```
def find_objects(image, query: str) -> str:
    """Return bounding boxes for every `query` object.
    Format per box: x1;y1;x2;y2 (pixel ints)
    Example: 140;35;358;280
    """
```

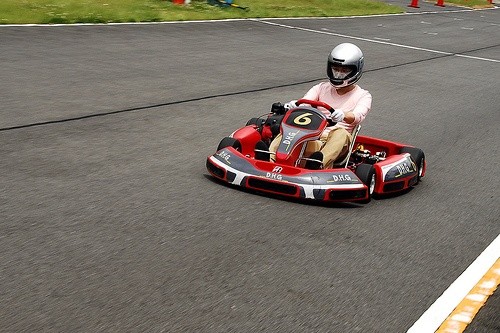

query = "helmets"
326;42;364;88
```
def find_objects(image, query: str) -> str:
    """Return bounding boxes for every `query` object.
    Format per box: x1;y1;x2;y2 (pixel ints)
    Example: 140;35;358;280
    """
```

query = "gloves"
328;108;345;122
288;99;304;108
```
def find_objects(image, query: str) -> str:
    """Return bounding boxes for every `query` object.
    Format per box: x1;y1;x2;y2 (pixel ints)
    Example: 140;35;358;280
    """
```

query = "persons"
254;43;371;170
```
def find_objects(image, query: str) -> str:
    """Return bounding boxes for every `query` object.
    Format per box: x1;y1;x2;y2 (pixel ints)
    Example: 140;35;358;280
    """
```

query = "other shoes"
255;140;270;161
305;151;323;169
